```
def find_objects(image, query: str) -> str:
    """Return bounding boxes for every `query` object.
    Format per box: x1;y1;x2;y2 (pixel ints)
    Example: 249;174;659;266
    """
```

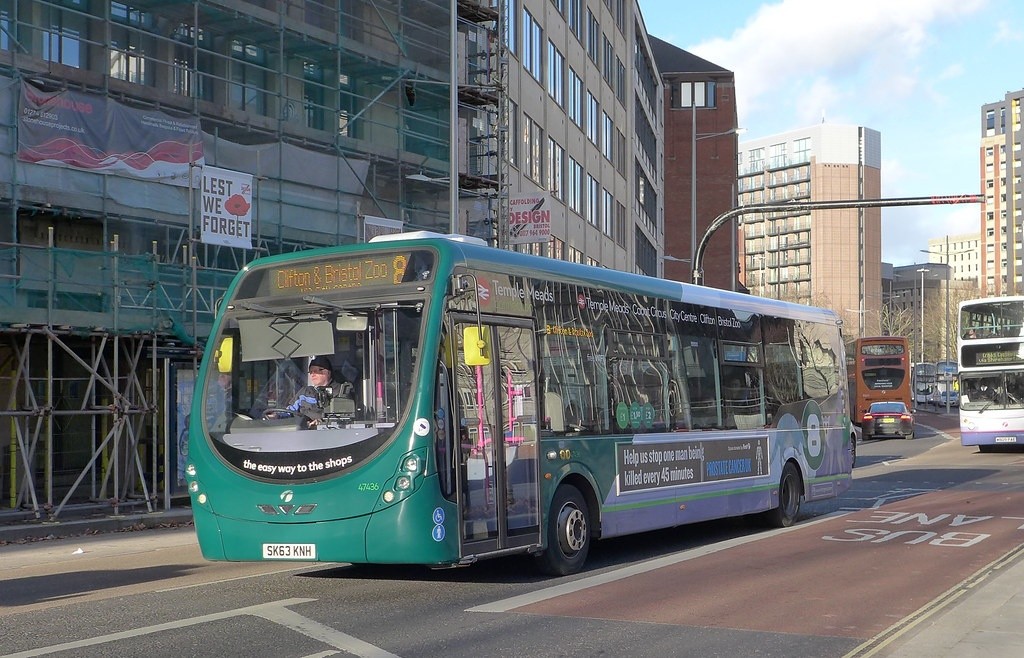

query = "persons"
975;382;995;401
263;357;358;427
961;326;976;338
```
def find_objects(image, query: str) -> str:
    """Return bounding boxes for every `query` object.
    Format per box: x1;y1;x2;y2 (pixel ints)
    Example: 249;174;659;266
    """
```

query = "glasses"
307;368;326;374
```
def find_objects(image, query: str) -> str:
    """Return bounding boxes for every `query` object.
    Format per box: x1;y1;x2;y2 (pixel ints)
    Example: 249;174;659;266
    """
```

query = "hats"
308;356;333;371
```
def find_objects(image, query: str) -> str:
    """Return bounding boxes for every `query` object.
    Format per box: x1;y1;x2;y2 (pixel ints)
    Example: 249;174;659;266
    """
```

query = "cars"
861;401;914;440
938;391;959;408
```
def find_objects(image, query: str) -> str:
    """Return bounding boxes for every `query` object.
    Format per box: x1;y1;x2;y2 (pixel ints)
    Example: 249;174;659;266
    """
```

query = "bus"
911;362;936;403
956;295;1024;452
845;337;911;425
184;231;851;576
936;361;959;395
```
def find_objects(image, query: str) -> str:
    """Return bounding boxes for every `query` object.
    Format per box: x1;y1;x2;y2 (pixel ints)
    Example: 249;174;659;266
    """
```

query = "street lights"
920;235;974;414
